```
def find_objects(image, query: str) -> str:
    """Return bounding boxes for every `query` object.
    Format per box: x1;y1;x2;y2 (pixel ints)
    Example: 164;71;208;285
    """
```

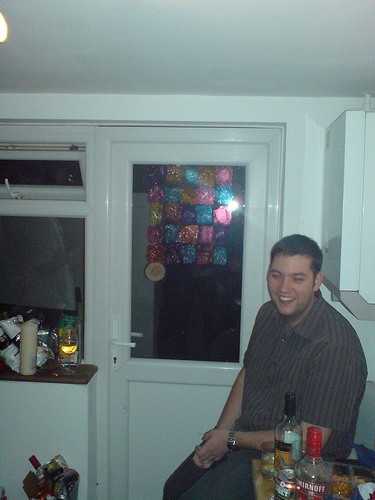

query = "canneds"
274;469;299;500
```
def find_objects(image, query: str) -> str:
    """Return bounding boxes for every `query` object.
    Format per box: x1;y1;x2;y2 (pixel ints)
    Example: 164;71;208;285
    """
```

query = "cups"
261;441;274;478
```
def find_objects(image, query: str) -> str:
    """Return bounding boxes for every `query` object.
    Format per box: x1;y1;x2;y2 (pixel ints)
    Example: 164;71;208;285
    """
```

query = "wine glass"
59;333;78;374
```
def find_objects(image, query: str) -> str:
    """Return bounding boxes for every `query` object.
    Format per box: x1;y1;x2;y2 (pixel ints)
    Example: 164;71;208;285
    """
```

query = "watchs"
227;430;236;452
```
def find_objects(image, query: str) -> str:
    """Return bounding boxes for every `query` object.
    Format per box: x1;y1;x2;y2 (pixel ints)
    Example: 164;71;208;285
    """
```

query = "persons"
161;233;369;500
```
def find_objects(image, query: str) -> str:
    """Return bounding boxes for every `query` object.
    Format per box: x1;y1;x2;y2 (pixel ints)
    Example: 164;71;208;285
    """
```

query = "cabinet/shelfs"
321;110;375;305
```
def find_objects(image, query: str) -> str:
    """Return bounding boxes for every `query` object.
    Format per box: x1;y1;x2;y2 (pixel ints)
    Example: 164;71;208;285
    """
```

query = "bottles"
295;427;332;500
273;392;303;488
